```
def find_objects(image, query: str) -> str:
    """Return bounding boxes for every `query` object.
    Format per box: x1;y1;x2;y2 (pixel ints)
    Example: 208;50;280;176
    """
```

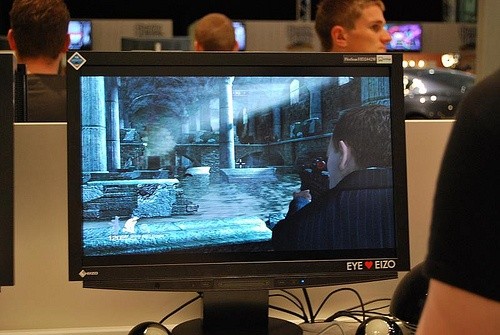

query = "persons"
315;0;392;52
8;0;71;122
193;13;238;51
413;72;500;335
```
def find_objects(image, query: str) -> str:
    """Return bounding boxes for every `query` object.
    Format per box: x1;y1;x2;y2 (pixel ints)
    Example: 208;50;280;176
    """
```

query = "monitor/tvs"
65;50;411;335
231;20;248;51
383;23;423;52
67;18;93;50
